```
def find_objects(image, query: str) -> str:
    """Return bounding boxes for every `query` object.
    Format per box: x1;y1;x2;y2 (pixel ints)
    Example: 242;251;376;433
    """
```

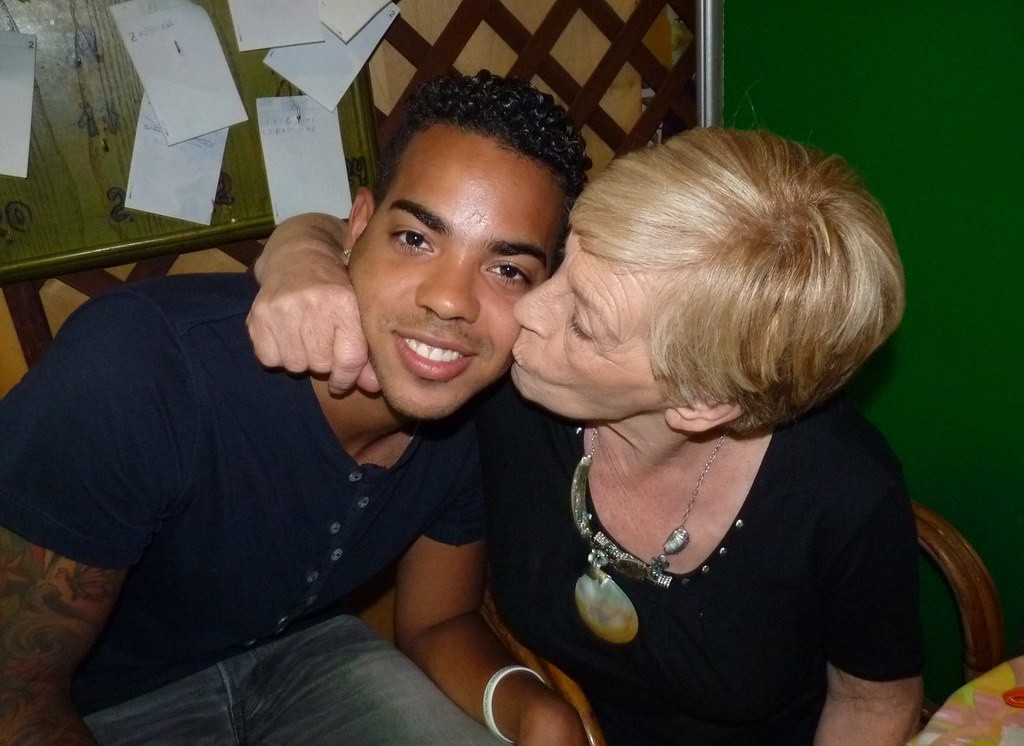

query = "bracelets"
481;663;548;744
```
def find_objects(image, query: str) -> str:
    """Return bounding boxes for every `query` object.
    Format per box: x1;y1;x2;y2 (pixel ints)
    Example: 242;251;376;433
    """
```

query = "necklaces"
568;423;732;646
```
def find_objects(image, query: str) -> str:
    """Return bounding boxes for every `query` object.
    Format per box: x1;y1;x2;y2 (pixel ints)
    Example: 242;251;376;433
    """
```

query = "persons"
0;68;596;746
244;122;928;746
